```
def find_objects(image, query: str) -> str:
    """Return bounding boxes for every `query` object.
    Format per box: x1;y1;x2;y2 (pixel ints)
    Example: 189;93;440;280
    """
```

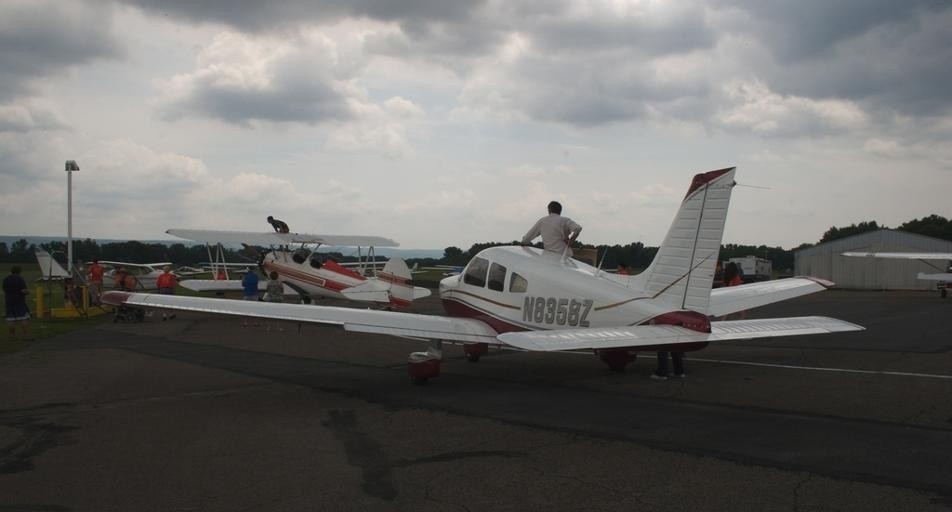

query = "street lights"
66;160;80;275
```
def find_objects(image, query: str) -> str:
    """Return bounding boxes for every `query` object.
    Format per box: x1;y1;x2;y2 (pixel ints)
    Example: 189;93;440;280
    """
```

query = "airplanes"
36;251;177;290
99;166;866;386
167;229;431;310
842;251;952;297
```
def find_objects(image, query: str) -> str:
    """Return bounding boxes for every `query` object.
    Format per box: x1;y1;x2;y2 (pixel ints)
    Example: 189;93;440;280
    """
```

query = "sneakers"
670;372;685;378
650;374;669;381
161;313;175;321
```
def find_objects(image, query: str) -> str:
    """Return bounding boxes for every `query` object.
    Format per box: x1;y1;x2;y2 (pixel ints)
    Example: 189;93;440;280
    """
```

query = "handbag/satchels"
262;291;270;302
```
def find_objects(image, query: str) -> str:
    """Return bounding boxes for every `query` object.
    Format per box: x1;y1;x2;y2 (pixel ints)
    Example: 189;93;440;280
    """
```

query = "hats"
247;266;255;271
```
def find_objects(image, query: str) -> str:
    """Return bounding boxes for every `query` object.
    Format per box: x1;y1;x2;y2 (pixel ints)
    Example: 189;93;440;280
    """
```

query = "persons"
648;344;686;381
241;263;262;327
267;215;290;253
265;269;286;332
722;261;745;320
113;267;135;317
2;264;35;342
521;200;583;261
87;256;104;306
156;266;178;320
614;262;627;274
